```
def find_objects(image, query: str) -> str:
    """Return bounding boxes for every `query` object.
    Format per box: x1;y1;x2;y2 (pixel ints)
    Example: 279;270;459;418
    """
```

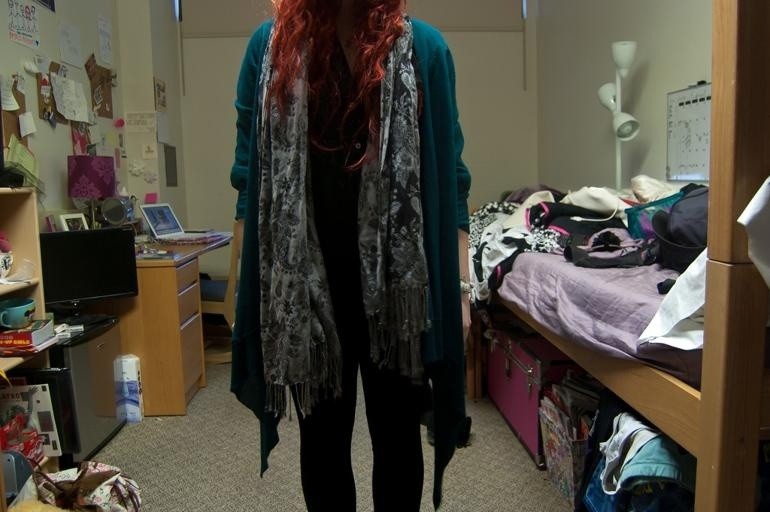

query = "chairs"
202;219;246;367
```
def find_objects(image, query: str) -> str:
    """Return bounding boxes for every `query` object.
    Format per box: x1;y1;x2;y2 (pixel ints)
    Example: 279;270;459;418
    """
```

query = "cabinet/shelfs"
0;185;85;512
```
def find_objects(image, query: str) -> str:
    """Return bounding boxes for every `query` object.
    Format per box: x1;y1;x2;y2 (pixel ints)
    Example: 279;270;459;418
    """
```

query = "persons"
226;1;472;512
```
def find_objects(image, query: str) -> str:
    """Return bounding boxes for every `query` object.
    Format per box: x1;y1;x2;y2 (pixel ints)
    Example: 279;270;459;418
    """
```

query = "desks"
90;233;231;418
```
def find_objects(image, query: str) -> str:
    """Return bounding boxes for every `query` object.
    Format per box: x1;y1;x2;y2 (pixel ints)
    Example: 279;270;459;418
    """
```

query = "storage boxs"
482;323;600;512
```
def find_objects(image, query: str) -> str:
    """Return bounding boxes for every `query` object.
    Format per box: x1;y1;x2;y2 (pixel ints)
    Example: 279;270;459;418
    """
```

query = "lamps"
597;40;638;192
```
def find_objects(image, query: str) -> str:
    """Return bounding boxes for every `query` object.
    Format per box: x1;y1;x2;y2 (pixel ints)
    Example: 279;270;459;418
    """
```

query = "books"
0;335;59;353
120;354;145;422
0;319;55;346
114;357;127;423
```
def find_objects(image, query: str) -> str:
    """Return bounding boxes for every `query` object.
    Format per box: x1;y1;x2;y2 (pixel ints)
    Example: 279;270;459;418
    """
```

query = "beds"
455;195;770;512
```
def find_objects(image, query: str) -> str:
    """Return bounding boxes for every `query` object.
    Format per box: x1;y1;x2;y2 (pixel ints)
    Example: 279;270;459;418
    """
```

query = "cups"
0;297;36;330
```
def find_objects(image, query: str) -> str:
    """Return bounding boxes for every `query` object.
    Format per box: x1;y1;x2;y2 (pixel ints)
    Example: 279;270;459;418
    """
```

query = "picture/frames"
45;211;90;233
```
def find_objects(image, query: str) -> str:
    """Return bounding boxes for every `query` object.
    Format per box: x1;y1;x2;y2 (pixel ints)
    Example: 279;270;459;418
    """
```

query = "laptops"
139;203;208;241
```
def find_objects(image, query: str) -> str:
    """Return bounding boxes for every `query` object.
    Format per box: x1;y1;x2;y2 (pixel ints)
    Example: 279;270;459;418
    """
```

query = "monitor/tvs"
40;227;138;326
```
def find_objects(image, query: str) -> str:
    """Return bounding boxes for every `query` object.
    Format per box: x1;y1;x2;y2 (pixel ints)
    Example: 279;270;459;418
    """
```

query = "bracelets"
460;278;473;294
